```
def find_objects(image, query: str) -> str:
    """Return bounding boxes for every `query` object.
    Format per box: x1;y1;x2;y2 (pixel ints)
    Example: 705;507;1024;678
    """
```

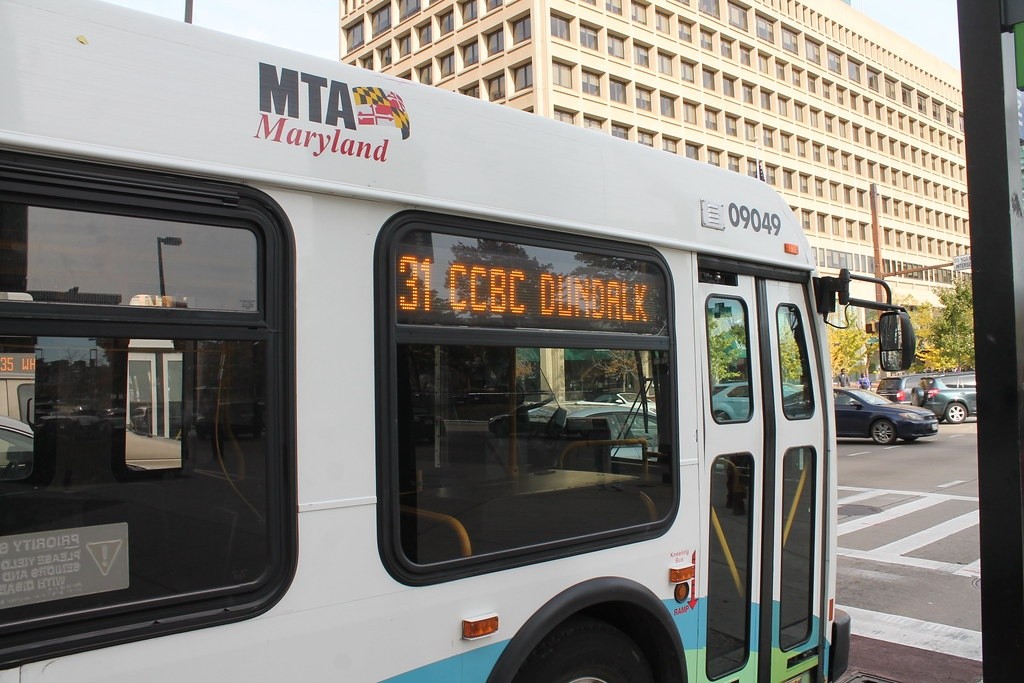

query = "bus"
0;339;185;469
2;0;916;681
515;307;749;404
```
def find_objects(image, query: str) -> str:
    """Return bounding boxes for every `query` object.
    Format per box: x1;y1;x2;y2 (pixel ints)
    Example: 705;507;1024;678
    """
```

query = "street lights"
156;235;182;298
36;347;43;359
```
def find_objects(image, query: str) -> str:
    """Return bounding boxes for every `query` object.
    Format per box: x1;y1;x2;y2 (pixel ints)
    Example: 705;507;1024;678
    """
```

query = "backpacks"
860;378;869;390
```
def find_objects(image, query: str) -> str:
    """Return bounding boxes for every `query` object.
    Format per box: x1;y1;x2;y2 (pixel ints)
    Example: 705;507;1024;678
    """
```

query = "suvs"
910;371;977;424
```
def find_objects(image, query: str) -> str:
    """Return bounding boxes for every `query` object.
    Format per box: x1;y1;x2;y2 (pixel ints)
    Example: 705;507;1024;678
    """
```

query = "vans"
875;372;940;405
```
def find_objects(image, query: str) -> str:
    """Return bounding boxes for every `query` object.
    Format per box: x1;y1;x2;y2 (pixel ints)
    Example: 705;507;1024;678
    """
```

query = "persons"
859;373;870;390
837;368;850;387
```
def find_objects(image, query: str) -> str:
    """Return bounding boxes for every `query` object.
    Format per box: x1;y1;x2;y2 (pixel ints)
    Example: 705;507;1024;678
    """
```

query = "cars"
735;465;749;474
715;458;730;472
131;406;152;428
146;407;182;432
488;402;547;436
408;408;446;443
527;393;659;462
193;386;267;439
782;386;938;444
596;393;657;413
711;382;799;423
36;402;126;431
1;416;152;472
